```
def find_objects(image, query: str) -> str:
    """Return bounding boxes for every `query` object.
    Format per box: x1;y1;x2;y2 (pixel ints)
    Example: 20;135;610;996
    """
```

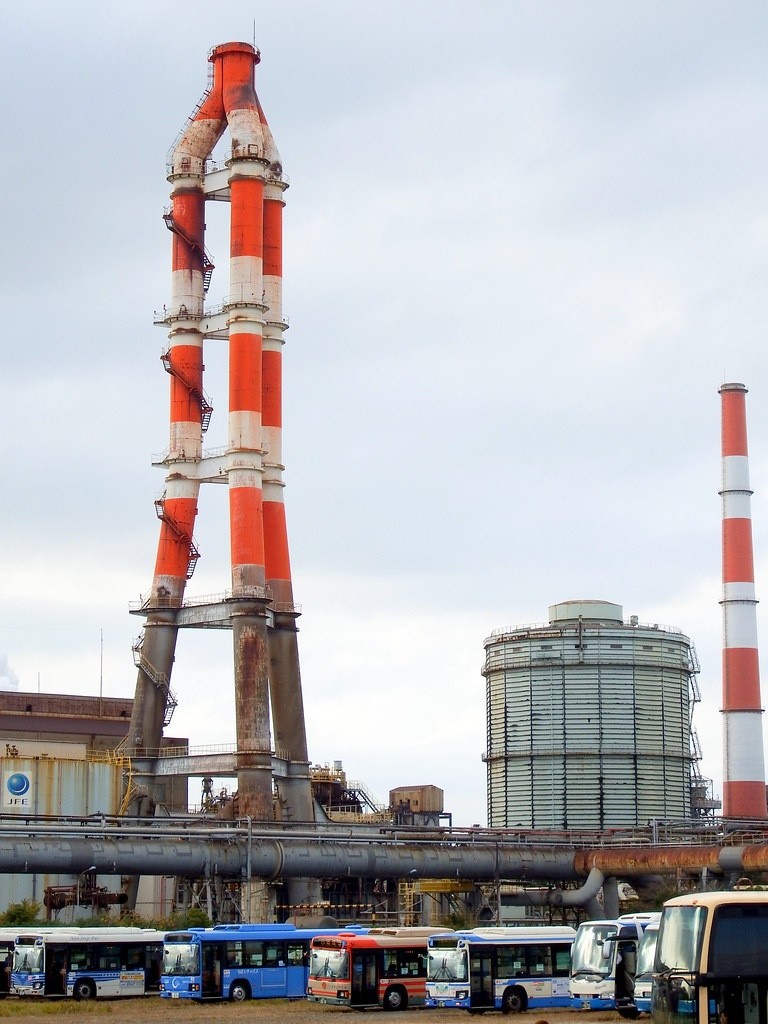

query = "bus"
155;924;371;1003
4;931;194;1002
630;919;717;1017
417;925;577;1016
303;926;456;1012
651;889;768;1023
567;912;662;1019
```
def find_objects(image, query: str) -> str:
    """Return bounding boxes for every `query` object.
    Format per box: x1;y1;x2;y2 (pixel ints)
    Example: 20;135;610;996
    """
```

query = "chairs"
387;964;396;977
